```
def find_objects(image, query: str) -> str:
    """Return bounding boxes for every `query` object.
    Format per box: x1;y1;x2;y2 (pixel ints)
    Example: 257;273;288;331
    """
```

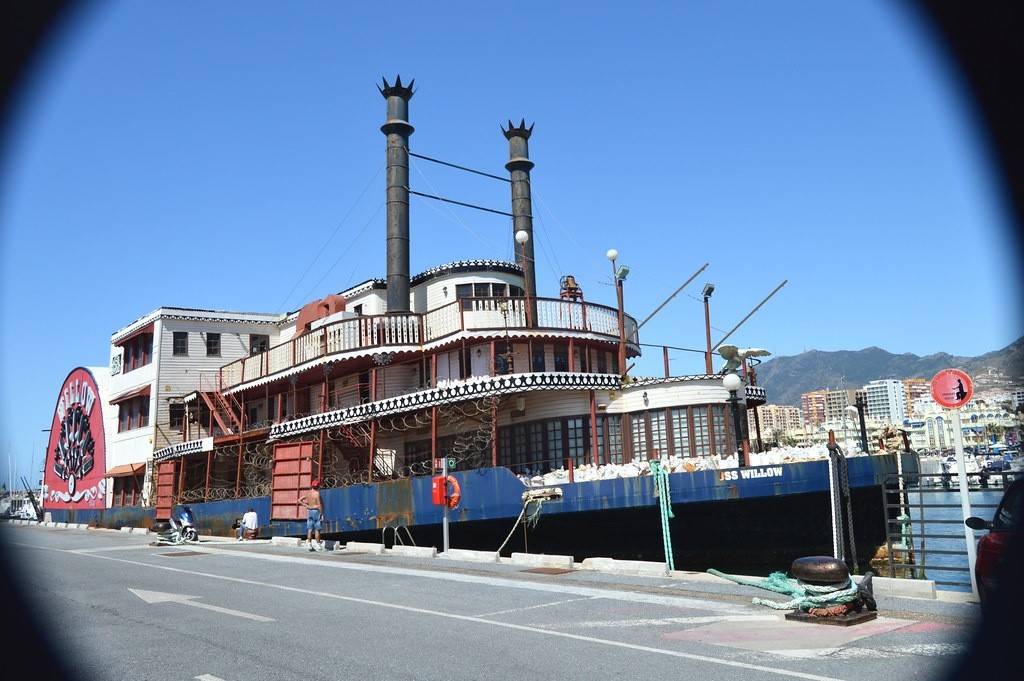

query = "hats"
312;481;319;487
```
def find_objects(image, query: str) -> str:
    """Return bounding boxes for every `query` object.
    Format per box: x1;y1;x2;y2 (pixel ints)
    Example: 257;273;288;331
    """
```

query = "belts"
309;508;318;510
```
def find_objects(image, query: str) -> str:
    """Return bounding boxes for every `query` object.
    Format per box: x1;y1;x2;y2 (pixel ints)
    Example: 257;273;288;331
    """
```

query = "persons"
177;498;187;505
296;480;325;553
237;507;258;541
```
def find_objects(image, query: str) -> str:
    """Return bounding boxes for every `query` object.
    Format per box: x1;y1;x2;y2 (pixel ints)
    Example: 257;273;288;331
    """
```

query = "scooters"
153;494;199;542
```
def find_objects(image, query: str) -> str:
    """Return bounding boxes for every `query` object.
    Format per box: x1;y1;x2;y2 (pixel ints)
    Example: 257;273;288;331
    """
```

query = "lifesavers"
447;476;462;508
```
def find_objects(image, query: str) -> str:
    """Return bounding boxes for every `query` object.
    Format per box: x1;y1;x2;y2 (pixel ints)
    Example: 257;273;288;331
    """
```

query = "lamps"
516;392;527;412
643;391;648;401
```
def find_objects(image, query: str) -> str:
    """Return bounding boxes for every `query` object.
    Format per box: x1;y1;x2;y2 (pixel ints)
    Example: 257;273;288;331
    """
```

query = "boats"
40;77;920;584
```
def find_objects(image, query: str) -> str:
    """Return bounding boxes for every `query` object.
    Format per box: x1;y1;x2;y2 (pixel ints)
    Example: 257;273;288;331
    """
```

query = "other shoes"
309;548;315;551
